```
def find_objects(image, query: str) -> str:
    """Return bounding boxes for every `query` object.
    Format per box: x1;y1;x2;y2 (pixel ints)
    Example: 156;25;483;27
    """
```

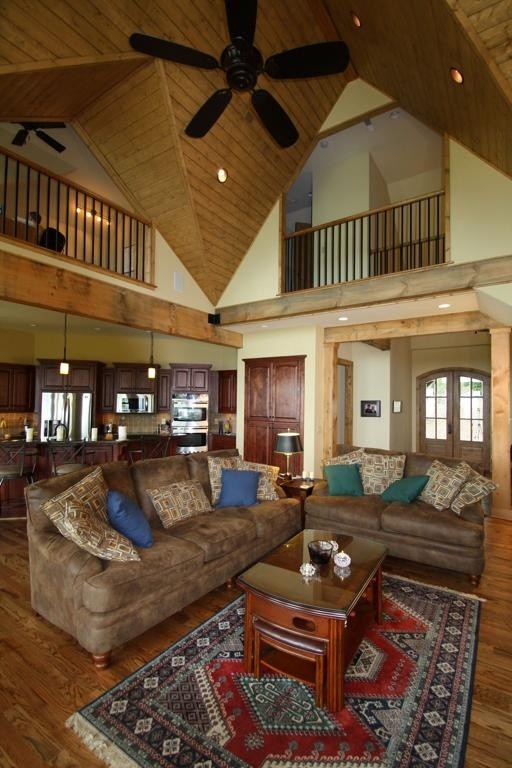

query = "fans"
126;0;351;147
6;119;70;152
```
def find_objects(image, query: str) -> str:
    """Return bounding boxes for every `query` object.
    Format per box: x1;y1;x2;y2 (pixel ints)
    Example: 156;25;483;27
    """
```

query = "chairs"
0;430;175;518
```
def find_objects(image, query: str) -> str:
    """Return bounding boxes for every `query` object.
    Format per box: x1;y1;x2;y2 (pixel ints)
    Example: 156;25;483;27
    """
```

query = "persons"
365;404;371;413
212;416;235;435
371;406;376;413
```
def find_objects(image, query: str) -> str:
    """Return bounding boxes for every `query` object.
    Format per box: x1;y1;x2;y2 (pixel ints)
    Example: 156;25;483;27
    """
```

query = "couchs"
304;444;492;584
24;447;304;672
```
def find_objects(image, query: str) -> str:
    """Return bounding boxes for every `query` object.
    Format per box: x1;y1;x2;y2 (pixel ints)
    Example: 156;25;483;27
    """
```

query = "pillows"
38;466;110;542
382;471;430;503
217;466;263;506
64;498;139;561
323;463;367;495
108;490;156;548
237;460;282;501
143;477;214;528
319;445;368;480
203;454;243;504
421;458;471;509
448;459;499;516
358;451;406;494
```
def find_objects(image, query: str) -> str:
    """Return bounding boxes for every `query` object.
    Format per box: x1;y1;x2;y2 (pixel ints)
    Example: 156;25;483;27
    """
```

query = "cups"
307;540;332;566
309;472;314;481
301;472;307;481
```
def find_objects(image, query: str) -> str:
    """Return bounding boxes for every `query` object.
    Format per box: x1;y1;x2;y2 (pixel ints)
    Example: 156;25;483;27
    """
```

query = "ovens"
171;401;209;455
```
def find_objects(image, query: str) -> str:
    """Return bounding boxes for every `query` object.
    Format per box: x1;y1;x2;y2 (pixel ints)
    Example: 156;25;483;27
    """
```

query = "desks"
275;476;319;504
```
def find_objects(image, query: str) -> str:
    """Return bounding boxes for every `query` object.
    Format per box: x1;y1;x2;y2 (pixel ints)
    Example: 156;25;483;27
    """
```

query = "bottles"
333;564;351;581
223;417;232;435
119;416;128;433
334;550;352;569
156;418;170;435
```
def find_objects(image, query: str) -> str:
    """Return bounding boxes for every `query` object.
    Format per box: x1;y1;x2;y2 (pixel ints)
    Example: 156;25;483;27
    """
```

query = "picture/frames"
392;399;403;414
360;399;381;417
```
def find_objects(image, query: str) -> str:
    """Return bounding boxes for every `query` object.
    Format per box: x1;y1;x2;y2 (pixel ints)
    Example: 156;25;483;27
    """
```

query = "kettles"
55;425;69;441
104;423;112;433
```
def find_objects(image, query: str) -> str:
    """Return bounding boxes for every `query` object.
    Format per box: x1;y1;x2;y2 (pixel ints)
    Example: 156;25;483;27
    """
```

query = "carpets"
66;569;490;768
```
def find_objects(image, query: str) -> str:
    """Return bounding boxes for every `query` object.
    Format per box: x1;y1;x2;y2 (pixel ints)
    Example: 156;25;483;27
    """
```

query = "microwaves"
113;393;154;414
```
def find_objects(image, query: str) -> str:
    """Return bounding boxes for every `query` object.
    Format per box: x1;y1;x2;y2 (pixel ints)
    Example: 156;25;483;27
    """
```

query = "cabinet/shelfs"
157;368;169;411
168;361;213;392
0;362;34;410
207;369;237;413
100;368;113;409
113;360;161;393
35;358;106;391
210;436;235;450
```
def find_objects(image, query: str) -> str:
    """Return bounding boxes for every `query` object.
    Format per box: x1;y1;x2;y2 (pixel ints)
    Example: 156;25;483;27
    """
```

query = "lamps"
146;331;156;378
274;428;303;480
58;312;71;375
